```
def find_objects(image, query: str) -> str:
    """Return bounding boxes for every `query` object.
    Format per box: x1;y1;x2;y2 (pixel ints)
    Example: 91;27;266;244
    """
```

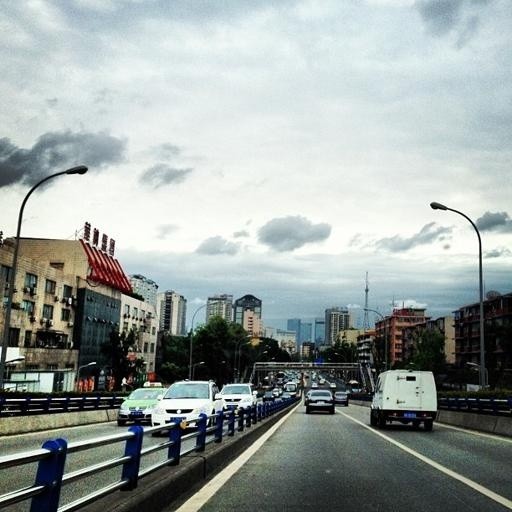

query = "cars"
118;370;348;434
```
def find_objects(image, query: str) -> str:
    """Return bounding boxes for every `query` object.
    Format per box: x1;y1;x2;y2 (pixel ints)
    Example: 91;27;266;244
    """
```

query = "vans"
370;369;438;429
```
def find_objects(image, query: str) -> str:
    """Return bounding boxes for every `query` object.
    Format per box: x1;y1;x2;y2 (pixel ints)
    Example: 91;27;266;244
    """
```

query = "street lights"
364;309;387;370
234;335;253;383
76;362;96;392
0;165;87;391
189;300;225;380
430;202;488;393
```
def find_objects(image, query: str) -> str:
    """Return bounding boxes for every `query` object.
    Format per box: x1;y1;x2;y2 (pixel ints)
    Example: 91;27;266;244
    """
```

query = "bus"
346;380;362;393
